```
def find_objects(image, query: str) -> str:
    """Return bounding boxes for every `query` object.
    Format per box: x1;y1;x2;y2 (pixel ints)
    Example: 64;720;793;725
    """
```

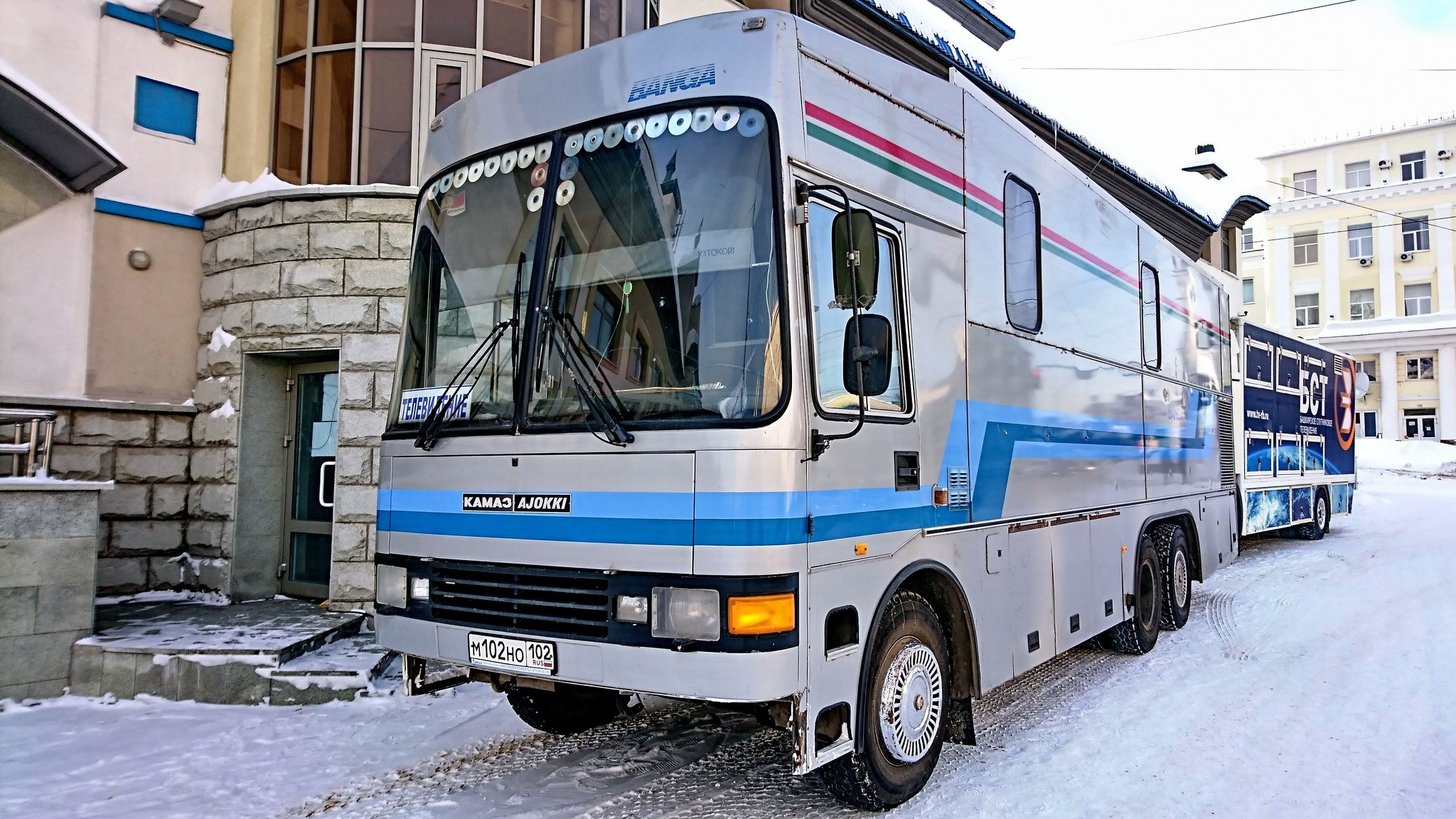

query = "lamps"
158;0;204;25
1238;311;1248;317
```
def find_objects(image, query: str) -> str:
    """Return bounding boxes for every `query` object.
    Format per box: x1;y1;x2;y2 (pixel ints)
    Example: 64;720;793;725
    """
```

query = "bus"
374;4;1239;814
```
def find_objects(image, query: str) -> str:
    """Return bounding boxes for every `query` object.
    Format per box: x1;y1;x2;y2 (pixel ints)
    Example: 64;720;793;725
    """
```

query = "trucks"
1229;317;1358;540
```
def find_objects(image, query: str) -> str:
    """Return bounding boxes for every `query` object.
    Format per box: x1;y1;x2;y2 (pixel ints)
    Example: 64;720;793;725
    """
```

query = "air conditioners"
1438;149;1452;159
1378;159;1392;169
1359;256;1371;266
1399;251;1413;261
